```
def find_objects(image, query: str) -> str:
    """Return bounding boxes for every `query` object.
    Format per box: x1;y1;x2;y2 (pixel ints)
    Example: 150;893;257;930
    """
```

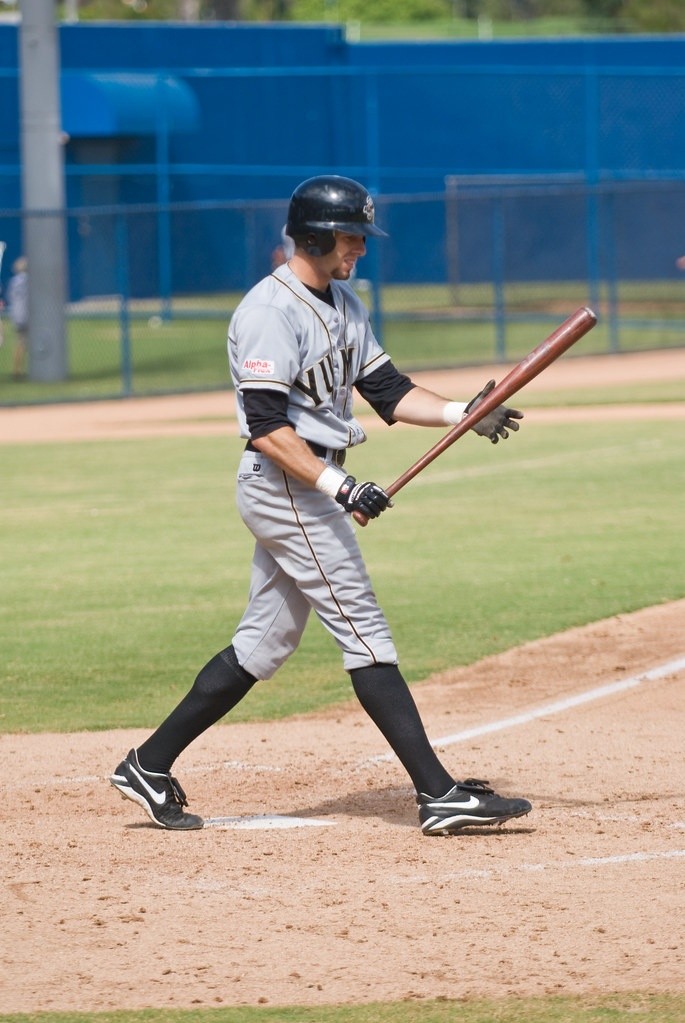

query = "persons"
109;175;533;836
6;257;28;379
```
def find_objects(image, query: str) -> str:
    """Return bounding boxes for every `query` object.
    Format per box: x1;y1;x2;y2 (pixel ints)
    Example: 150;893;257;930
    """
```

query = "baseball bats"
352;305;599;529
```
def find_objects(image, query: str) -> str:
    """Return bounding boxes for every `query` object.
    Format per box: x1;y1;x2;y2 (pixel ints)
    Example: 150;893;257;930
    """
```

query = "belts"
244;437;346;467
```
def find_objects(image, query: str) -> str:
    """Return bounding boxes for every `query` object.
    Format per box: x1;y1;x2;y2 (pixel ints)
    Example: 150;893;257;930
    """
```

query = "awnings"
60;72;203;138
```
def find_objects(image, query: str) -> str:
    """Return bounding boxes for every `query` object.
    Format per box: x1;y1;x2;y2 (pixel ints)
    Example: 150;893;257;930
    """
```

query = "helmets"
285;174;388;255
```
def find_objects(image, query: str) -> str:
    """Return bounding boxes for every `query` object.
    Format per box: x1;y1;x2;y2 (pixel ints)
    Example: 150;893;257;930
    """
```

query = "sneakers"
415;776;532;834
109;748;206;830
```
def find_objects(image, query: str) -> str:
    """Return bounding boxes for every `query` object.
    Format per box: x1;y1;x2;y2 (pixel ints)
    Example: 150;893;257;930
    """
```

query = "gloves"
336;476;395;519
461;380;525;443
